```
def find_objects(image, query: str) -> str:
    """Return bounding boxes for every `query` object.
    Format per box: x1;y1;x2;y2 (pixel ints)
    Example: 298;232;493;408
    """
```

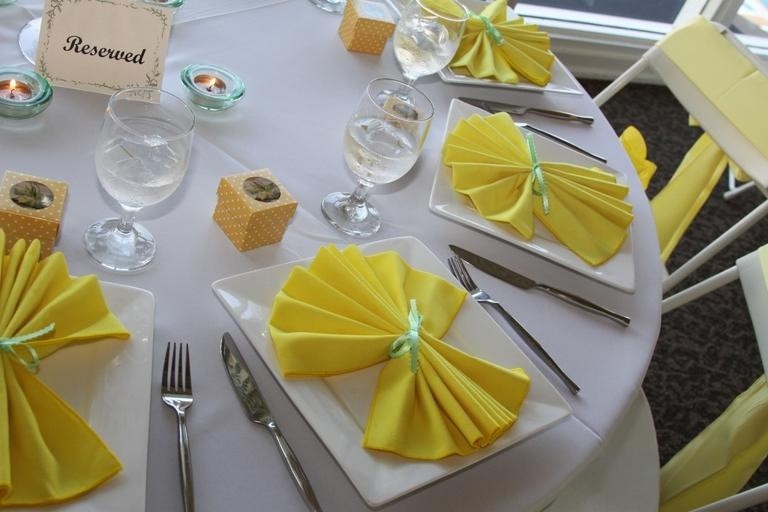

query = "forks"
450;258;581;398
160;340;195;511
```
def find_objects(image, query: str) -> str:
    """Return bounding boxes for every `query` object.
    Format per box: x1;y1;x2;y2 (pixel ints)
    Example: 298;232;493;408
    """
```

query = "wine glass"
319;77;434;240
84;88;194;270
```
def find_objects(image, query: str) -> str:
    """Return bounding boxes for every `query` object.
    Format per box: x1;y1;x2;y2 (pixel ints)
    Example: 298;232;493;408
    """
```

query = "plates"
438;17;583;93
210;237;572;508
430;99;639;299
0;274;155;511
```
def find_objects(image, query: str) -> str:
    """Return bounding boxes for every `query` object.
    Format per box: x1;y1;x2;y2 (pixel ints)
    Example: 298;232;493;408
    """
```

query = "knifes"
219;333;324;512
458;95;597;125
448;240;637;328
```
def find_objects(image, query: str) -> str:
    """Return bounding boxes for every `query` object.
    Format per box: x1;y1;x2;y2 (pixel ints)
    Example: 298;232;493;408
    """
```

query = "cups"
394;2;466;81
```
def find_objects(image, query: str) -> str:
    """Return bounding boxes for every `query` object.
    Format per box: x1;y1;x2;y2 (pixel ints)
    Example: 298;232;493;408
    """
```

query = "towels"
445;114;633;267
267;243;531;459
0;241;128;506
424;0;554;87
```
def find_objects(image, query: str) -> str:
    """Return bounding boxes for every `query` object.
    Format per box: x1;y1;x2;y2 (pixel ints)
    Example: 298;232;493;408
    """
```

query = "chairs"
723;160;754;200
547;252;768;511
594;19;768;294
